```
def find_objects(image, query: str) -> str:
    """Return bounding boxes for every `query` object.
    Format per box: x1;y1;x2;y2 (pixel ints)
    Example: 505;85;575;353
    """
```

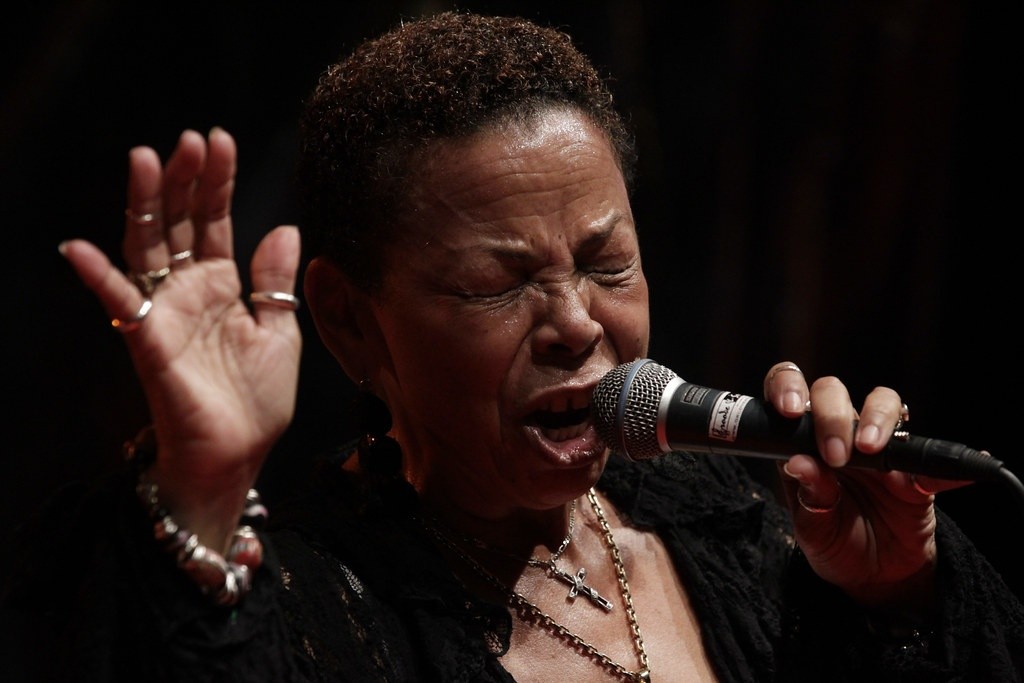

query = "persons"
58;11;1024;683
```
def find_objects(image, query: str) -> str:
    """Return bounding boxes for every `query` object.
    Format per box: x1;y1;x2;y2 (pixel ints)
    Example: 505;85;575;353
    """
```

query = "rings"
126;210;161;226
169;250;193;264
760;365;803;401
910;474;936;496
127;267;169;295
892;402;910;429
248;290;301;313
792;484;843;514
114;302;154;337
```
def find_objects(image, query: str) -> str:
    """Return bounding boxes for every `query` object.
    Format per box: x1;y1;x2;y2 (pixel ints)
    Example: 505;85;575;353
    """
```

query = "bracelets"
133;441;267;605
875;606;941;658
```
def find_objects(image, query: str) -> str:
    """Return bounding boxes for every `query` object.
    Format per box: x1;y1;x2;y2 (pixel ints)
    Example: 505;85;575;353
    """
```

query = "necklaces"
424;499;614;613
359;465;650;683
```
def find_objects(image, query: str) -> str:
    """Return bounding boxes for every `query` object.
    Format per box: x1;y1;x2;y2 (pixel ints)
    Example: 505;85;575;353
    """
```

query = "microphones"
589;358;1001;485
